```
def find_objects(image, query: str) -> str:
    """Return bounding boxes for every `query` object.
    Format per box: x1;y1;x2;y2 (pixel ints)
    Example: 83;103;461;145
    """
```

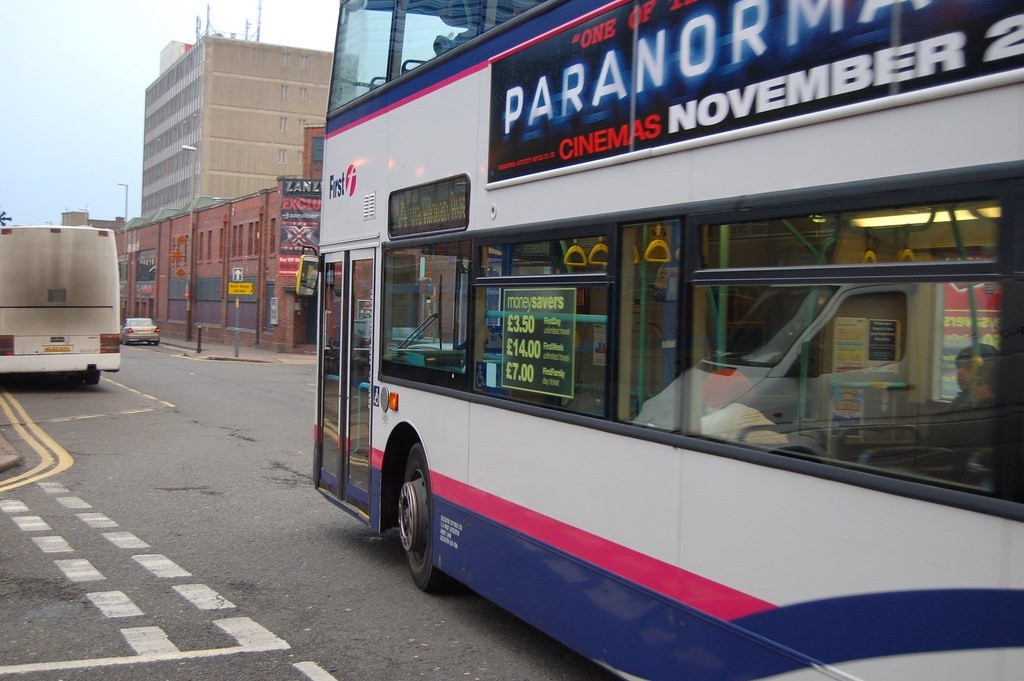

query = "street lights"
118;183;128;255
181;145;197;343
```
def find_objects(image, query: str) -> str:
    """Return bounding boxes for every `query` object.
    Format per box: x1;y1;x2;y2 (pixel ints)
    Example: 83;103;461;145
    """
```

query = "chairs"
856;446;963;482
738;424;827;458
835;425;920;462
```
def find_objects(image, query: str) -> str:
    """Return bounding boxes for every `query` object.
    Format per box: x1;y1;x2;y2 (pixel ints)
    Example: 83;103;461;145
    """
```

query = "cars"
120;318;161;347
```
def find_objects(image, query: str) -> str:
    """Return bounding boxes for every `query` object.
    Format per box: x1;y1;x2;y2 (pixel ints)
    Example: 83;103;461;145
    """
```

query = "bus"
295;0;1024;681
1;224;120;389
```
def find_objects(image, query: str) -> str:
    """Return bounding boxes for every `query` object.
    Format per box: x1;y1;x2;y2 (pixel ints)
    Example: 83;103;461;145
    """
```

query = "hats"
958;343;999;384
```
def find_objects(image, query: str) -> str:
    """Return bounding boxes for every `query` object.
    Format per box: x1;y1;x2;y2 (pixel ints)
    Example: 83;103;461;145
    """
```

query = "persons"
699;369;788;445
945;343;1002;410
